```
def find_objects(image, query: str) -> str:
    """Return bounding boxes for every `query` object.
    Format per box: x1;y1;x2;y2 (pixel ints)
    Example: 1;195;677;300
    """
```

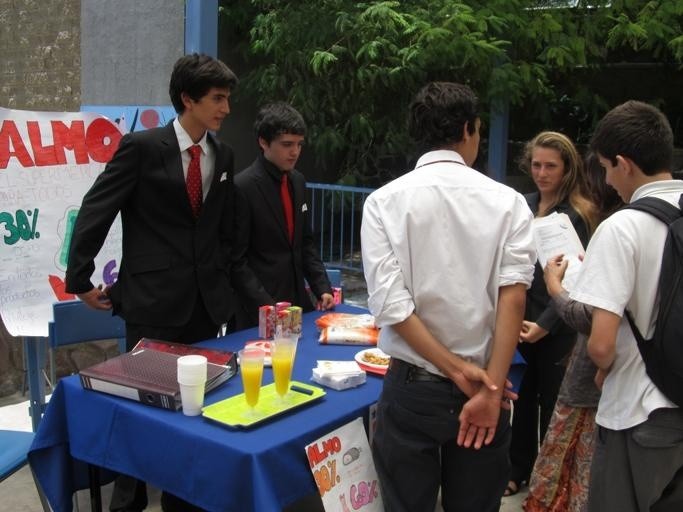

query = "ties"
186;145;203;215
282;174;293;246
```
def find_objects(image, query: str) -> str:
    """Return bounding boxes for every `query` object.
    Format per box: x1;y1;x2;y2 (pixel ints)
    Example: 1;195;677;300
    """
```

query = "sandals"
504;481;523;496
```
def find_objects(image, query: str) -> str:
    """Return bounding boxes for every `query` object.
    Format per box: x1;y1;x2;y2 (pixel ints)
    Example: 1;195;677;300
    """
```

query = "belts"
386;356;452;382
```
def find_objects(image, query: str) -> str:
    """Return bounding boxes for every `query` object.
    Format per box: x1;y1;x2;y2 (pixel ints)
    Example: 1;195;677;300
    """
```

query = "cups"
177;354;208;416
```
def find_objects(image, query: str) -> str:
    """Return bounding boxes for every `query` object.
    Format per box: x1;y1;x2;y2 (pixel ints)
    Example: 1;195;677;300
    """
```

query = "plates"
235;355;273;367
355;347;389;369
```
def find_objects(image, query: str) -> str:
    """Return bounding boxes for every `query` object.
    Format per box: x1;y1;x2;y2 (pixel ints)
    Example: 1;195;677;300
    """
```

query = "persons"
356;82;539;511
569;100;683;512
503;131;625;512
62;52;241;512
232;99;335;336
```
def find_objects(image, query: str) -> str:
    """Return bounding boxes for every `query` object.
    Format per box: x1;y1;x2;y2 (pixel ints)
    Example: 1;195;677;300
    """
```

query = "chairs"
49;299;127;511
0;430;52;512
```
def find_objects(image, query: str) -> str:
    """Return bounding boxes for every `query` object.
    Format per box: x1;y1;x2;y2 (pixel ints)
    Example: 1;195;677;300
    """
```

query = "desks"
28;305;529;512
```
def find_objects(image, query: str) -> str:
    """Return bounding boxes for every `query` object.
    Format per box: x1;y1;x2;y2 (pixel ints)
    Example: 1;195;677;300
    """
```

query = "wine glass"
239;348;264;419
272;335;300;410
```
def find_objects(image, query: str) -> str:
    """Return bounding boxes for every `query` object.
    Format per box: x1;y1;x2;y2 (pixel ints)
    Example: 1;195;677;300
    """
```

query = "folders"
77;346;234;413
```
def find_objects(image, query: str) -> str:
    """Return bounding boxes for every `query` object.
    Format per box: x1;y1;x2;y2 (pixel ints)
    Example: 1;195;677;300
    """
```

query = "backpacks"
619;194;682;408
